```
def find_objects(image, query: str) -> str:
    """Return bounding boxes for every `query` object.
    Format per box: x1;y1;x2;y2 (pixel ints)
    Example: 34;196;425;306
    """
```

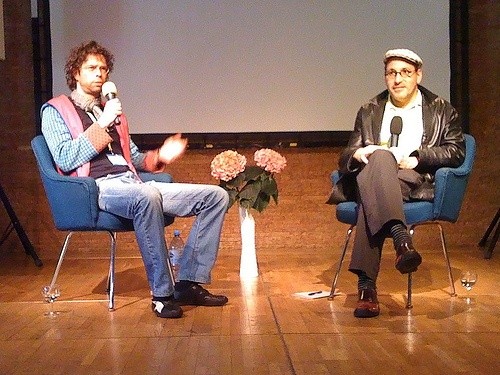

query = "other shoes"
174;282;228;306
151;296;184;318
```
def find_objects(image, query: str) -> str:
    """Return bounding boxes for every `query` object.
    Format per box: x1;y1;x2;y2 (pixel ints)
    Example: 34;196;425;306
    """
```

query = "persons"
39;42;229;319
324;48;467;318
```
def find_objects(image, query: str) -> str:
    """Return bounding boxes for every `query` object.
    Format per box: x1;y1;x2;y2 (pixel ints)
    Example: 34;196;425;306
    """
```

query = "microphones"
102;81;120;125
390;116;403;147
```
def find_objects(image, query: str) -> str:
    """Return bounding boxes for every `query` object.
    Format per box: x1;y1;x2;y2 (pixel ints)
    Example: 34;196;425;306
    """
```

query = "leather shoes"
394;243;422;274
354;288;380;317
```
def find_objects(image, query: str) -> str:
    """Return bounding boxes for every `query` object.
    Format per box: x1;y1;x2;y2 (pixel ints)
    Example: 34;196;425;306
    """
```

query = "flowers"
209;148;287;212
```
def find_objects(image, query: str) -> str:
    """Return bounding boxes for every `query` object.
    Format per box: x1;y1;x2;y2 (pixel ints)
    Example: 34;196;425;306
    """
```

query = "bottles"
167;230;185;281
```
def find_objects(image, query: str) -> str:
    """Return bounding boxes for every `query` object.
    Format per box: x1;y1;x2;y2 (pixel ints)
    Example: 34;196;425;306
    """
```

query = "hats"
383;48;423;68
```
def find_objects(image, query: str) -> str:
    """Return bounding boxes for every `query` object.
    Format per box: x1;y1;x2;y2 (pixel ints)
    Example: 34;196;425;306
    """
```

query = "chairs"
30;133;182;312
329;133;478;310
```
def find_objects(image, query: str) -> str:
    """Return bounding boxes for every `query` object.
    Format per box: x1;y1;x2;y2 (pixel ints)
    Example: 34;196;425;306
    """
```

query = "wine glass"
41;285;60;317
460;271;477;300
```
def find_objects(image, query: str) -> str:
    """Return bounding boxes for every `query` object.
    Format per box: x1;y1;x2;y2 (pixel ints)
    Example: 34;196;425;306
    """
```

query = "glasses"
82;65;110;74
384;68;415;78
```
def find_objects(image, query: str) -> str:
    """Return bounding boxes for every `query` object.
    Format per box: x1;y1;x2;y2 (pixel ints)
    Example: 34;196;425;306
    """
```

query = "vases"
238;200;259;278
